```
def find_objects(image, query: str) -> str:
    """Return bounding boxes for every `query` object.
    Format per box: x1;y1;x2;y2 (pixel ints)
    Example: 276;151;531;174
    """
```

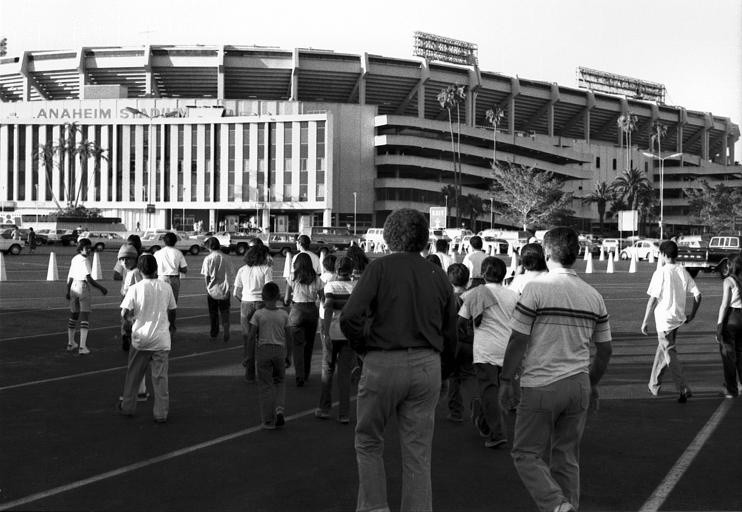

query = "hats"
116;243;138;261
335;255;356;277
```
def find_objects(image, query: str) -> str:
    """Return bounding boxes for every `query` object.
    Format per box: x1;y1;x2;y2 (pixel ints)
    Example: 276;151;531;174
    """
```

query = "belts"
366;345;429;352
164;274;180;277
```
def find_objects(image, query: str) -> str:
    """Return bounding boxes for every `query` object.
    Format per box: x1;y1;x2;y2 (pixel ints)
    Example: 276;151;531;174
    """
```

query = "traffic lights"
146;207;155;213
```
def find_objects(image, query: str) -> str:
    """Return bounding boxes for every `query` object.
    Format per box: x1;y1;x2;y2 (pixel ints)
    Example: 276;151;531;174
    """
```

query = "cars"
0;223;129;256
138;226;366;253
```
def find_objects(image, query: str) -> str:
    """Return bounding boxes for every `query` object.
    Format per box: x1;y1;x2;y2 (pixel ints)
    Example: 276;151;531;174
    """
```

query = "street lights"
641;151;684;238
35;184;39;222
489;197;494;229
125;105;179;228
267;187;272;231
445;195;449;228
170;184;175;229
139;186;146;228
255;189;259;229
183;185;186;232
352;192;357;236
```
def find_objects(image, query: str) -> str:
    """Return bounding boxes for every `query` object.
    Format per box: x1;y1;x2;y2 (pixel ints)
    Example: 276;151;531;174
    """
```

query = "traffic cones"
584;252;596;275
0;252;7;282
44;251;59;282
89;251;104;281
627;253;637;274
510;253;518;274
604;252;614;274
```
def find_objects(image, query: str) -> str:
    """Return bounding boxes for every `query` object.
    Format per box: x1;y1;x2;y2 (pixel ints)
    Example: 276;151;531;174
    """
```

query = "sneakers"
677;387;692;404
484;432;508;449
79;346;90;355
118;390;151;402
475;415;490;438
296;377;305;389
315;408;329;418
264;420;276;430
445;414;465;423
121;333;131;352
240;376;256;384
350;366;363;387
336;414;349;423
274;406;285;427
66;342;78;352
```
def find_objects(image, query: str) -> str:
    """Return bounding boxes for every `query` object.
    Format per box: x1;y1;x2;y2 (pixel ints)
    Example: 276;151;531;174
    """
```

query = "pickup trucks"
673;236;742;279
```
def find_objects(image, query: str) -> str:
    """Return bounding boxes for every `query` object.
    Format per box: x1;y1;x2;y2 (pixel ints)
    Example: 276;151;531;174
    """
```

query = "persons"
11;227;21;240
27;227;37;254
716;253;742;400
640;241;702;404
